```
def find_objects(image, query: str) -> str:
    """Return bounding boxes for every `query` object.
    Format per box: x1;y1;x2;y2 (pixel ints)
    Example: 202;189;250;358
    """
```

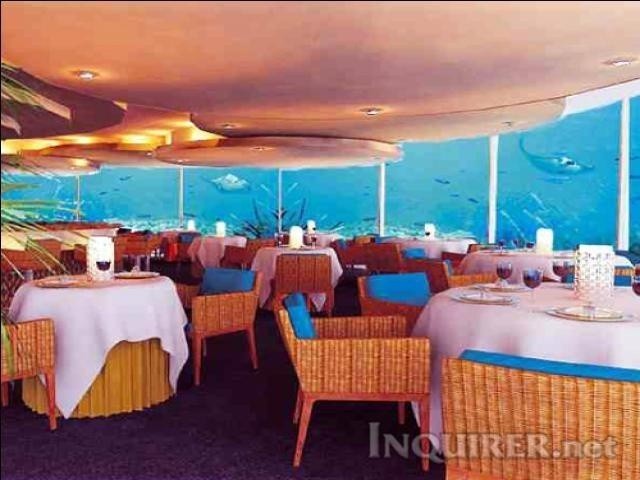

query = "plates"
460;293;512;302
114;271;160;278
552;303;624;319
35;277;92;288
479;282;526;292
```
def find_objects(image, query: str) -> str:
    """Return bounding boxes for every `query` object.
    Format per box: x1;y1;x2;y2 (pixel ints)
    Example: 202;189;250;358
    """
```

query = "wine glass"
522;268;544;312
96;258;112;283
497;263;513;289
553;261;571;289
631;264;640;324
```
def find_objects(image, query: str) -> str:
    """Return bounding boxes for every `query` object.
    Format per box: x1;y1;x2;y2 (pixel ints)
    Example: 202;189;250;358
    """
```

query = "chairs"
357;271;432;336
1;319;58;433
439;348;640;480
273;290;432;472
176;266;264;386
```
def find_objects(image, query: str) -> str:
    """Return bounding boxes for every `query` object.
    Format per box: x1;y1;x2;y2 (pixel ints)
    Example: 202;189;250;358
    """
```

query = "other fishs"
137;214;151;218
468;198;480;203
184;213;196;218
539;177;574;184
362;216;376;220
449;194;460;198
1;178;41;195
201;172;253;194
112;188;120;191
433;176;450;184
519;134;594;176
98;191;108;195
120;175;132;181
